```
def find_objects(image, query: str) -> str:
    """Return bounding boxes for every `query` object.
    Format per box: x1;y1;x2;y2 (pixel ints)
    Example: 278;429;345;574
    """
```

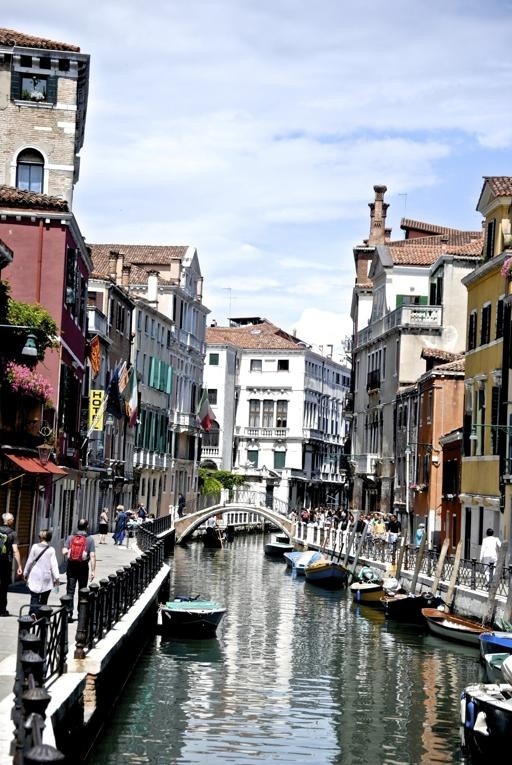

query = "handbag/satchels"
25;560;38;581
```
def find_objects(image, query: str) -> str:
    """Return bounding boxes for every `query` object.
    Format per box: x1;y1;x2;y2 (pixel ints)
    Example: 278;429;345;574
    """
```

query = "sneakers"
0;610;73;625
98;541;122;545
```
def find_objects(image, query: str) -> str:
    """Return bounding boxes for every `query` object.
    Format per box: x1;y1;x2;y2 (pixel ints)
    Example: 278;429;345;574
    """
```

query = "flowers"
5;359;55;401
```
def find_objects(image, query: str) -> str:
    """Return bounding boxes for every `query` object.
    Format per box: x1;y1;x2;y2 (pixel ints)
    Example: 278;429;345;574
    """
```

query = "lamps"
0;325;39;356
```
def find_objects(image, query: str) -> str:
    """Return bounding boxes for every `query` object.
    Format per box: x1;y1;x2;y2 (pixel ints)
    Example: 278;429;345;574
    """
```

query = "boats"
157;592;227;640
202;526;228;547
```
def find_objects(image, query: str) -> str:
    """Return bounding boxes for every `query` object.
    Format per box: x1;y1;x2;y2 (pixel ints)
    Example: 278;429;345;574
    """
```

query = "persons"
62;519;96;622
0;513;23;616
178;493;185;518
207;515;217;529
289;504;427;560
23;529;60;623
480;528;502;587
99;503;148;545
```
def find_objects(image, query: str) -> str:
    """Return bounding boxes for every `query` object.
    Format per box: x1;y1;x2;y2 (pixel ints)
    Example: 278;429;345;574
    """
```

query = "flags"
197;390;216;435
88;336;138;430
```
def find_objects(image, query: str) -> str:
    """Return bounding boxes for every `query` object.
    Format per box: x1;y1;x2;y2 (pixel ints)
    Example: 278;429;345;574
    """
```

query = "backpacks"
66;532;90;562
0;528;14;563
